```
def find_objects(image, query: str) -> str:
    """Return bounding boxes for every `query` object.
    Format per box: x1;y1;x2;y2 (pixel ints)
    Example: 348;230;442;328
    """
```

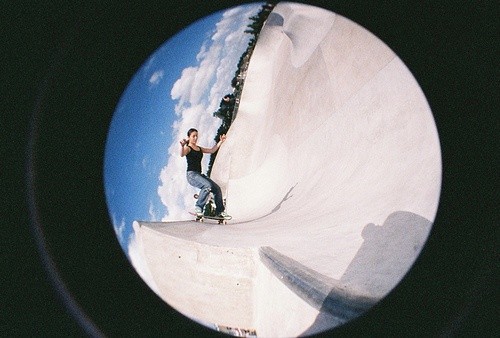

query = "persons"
179;128;230;220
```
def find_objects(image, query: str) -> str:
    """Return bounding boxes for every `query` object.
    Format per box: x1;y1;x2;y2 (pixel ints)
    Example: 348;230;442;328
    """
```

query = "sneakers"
196;210;204;217
215;213;226;219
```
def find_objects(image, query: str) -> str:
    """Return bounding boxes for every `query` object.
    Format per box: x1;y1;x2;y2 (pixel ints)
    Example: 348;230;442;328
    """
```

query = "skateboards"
188;211;232;225
